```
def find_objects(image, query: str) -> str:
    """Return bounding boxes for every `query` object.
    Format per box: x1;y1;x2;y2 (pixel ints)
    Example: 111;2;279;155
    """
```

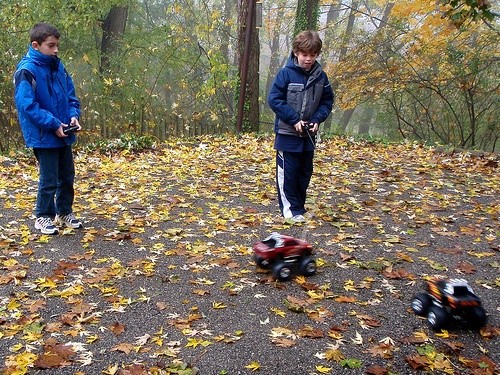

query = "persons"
268;30;334;224
14;22;83;231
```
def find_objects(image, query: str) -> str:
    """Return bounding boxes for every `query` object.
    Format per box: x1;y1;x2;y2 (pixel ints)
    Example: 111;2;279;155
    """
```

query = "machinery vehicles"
413;276;487;330
253;234;318;282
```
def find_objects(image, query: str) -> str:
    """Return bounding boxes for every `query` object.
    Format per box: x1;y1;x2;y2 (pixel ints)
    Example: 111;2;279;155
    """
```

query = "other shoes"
291;215;306;223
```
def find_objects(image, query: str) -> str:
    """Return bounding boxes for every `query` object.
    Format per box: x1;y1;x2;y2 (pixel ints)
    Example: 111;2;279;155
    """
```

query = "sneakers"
34;217;59;235
55;212;83;229
